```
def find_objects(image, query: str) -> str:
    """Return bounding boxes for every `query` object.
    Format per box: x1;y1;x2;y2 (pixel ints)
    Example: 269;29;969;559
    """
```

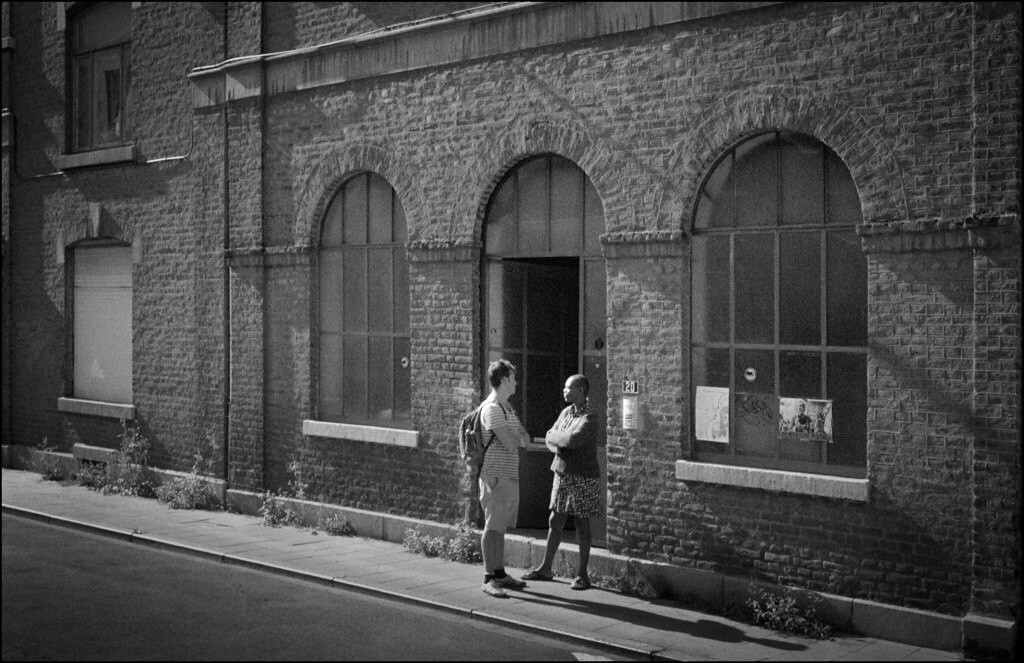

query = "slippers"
521;570;553;581
571;577;591;590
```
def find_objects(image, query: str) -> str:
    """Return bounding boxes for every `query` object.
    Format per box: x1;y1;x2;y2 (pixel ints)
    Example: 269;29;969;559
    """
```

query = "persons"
521;374;602;590
478;358;531;598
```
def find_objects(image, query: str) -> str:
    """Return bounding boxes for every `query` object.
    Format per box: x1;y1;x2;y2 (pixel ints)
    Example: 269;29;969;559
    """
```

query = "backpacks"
459;399;507;467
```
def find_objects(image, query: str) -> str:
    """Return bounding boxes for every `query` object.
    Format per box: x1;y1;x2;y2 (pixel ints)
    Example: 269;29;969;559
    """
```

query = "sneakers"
481;574;527;598
